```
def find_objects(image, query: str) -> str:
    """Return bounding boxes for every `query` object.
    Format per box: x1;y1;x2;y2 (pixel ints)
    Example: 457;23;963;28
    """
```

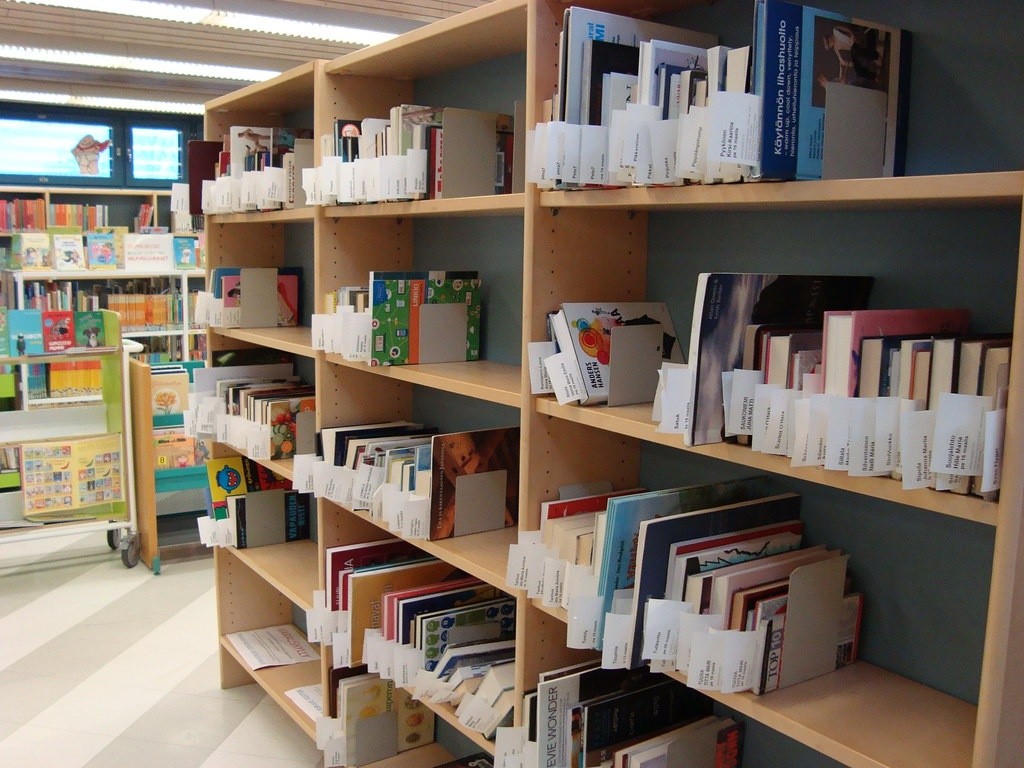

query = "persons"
823;26;883;82
816;72;849;89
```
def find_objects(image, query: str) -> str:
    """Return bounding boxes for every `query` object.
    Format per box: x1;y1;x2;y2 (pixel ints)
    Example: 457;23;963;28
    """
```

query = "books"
0;2;1011;768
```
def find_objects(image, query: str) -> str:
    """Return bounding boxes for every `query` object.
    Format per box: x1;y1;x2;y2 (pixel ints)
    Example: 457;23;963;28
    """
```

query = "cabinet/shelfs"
0;185;197;359
200;0;1024;768
0;309;145;569
129;355;213;575
0;267;207;488
